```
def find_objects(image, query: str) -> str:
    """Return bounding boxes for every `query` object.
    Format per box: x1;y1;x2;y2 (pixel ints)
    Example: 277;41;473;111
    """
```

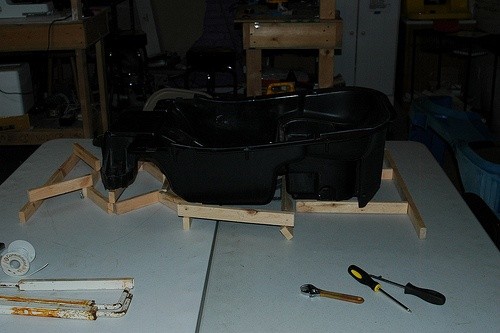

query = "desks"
0;138;217;333
195;137;500;333
231;11;345;97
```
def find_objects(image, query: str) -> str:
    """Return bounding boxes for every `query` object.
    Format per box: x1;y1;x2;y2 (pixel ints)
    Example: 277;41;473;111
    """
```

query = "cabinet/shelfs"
332;0;403;105
398;18;500;118
0;6;113;146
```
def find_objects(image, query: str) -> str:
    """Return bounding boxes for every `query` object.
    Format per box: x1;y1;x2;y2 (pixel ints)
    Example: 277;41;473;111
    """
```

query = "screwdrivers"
369;274;446;306
348;265;412;313
